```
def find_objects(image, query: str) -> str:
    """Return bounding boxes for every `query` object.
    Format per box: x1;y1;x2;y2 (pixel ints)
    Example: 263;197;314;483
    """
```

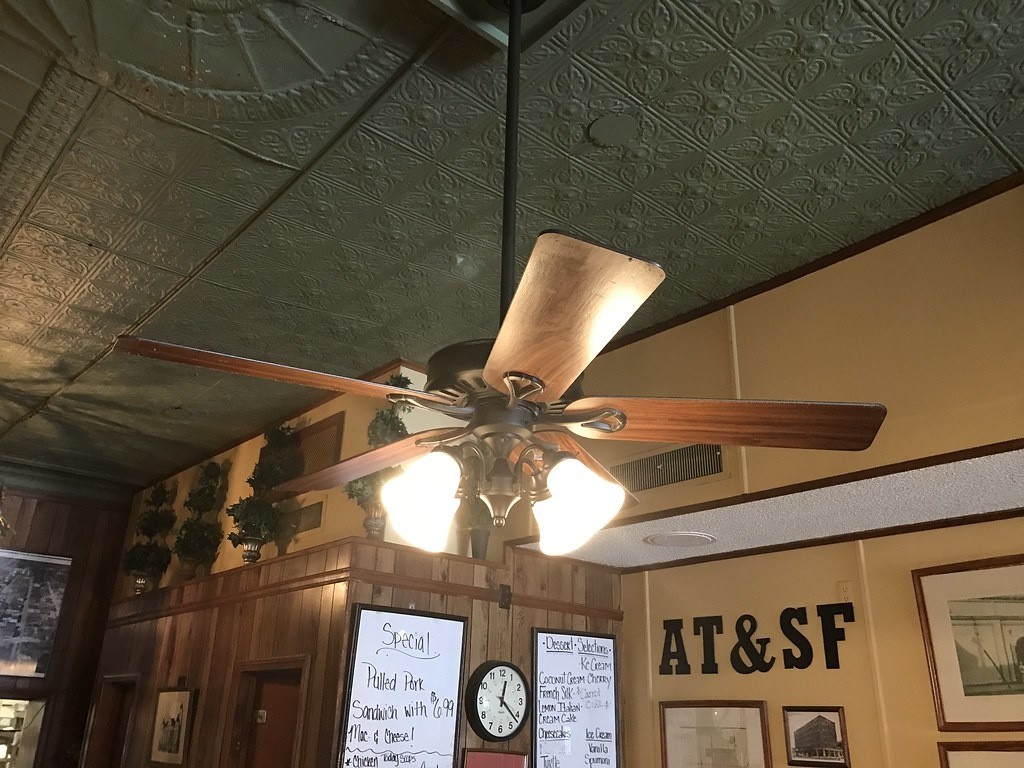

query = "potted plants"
226;422;295;564
121;476;170;595
343;372;415;540
175;461;219;581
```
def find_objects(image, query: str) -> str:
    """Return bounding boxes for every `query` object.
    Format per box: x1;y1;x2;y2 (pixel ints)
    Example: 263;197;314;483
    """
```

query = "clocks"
149;686;199;768
464;659;531;741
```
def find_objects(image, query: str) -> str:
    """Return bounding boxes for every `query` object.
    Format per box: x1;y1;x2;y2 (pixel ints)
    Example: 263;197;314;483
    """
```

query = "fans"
117;0;890;506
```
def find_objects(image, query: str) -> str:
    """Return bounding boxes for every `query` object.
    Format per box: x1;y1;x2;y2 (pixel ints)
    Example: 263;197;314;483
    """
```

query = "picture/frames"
910;554;1024;733
659;700;773;768
462;748;529;768
937;741;1024;768
781;705;851;768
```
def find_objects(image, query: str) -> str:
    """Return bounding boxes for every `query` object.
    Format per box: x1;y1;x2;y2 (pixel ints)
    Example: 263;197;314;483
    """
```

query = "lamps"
379;404;626;557
